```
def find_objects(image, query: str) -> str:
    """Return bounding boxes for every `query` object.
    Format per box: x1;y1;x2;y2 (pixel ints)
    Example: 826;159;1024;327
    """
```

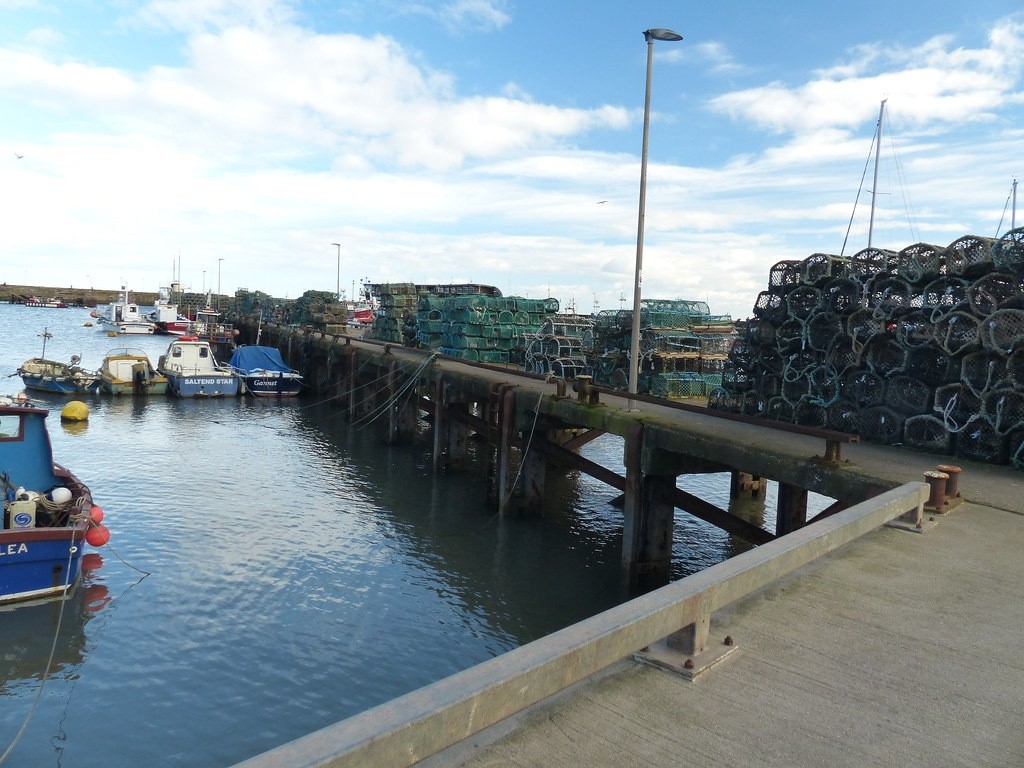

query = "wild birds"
15;153;24;159
596;200;609;205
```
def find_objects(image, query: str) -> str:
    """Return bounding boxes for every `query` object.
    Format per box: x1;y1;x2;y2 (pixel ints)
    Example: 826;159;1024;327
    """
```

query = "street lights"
202;270;208;294
218;258;224;312
331;243;341;300
628;29;684;412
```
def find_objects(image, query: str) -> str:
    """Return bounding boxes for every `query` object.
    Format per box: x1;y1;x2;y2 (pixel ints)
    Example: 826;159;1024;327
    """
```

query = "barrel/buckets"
10;501;36;530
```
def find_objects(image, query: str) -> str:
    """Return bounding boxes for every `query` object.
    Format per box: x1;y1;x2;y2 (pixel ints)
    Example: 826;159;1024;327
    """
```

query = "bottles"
15;487;25;501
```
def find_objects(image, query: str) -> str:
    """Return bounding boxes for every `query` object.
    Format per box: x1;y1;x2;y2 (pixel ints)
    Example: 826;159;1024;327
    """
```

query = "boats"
144;285;196;337
99;348;170;397
0;392;95;606
24;295;70;309
182;288;240;354
156;335;240;400
17;326;102;395
96;279;156;335
228;343;305;397
